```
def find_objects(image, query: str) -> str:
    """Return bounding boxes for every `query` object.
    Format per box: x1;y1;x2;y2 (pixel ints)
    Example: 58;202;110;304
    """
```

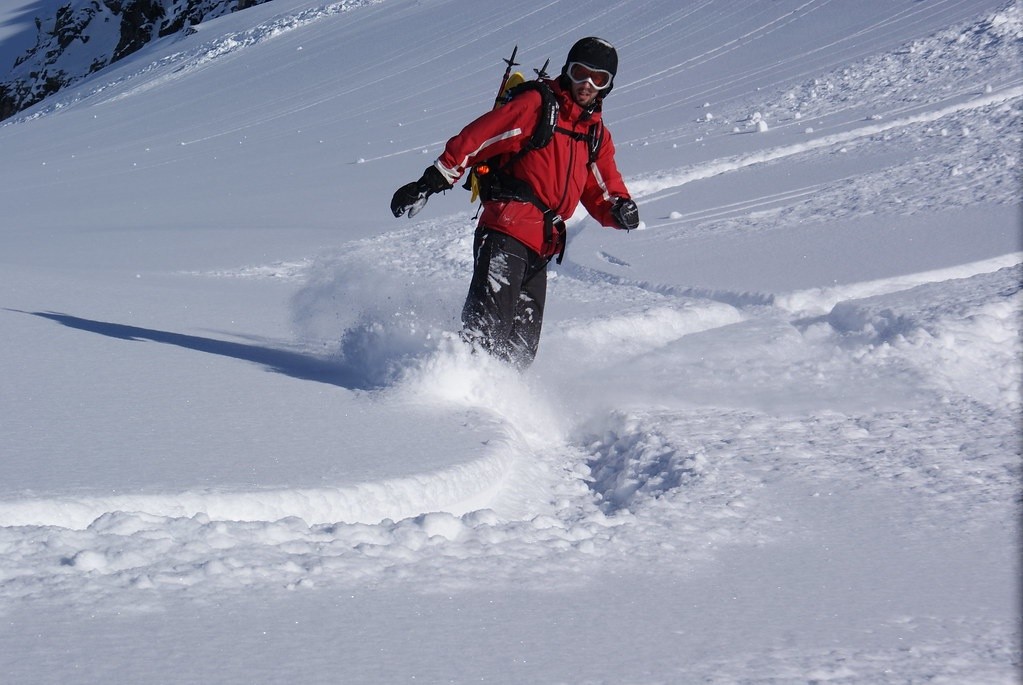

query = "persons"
391;37;640;372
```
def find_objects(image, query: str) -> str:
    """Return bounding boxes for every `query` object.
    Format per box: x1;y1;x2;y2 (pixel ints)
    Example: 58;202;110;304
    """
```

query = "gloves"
612;197;640;230
389;168;442;218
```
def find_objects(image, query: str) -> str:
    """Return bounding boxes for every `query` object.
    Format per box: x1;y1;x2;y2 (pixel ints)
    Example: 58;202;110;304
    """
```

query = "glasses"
566;62;612;90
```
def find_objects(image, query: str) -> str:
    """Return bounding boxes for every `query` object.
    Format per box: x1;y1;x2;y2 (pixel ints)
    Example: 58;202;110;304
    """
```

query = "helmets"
566;36;618;98
